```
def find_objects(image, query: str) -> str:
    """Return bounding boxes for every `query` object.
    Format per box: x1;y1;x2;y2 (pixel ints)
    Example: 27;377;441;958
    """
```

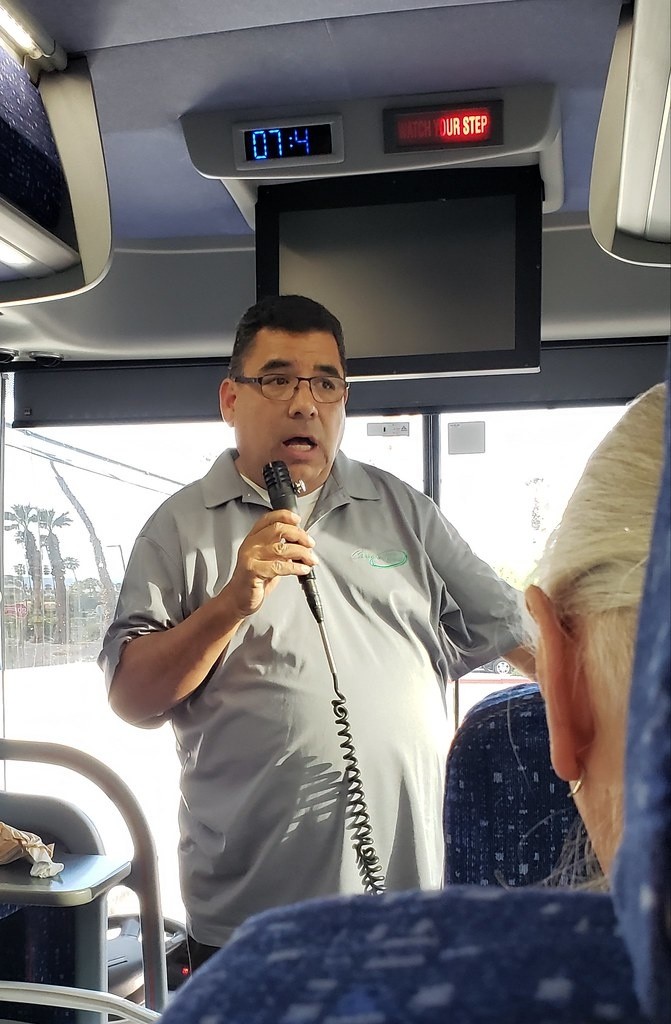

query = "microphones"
263;459;322;623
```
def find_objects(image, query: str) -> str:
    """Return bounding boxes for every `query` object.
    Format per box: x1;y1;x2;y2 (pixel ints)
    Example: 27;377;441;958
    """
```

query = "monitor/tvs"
256;166;541;381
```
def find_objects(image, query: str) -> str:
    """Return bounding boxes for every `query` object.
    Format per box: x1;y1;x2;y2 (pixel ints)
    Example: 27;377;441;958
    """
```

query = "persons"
521;378;670;894
92;293;548;976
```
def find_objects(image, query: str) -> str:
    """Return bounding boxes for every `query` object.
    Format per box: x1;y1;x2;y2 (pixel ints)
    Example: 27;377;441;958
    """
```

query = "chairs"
152;429;669;1021
437;676;606;888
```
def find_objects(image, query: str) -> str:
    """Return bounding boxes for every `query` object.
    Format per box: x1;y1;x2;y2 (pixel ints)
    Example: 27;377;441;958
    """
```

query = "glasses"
233;374;350;405
